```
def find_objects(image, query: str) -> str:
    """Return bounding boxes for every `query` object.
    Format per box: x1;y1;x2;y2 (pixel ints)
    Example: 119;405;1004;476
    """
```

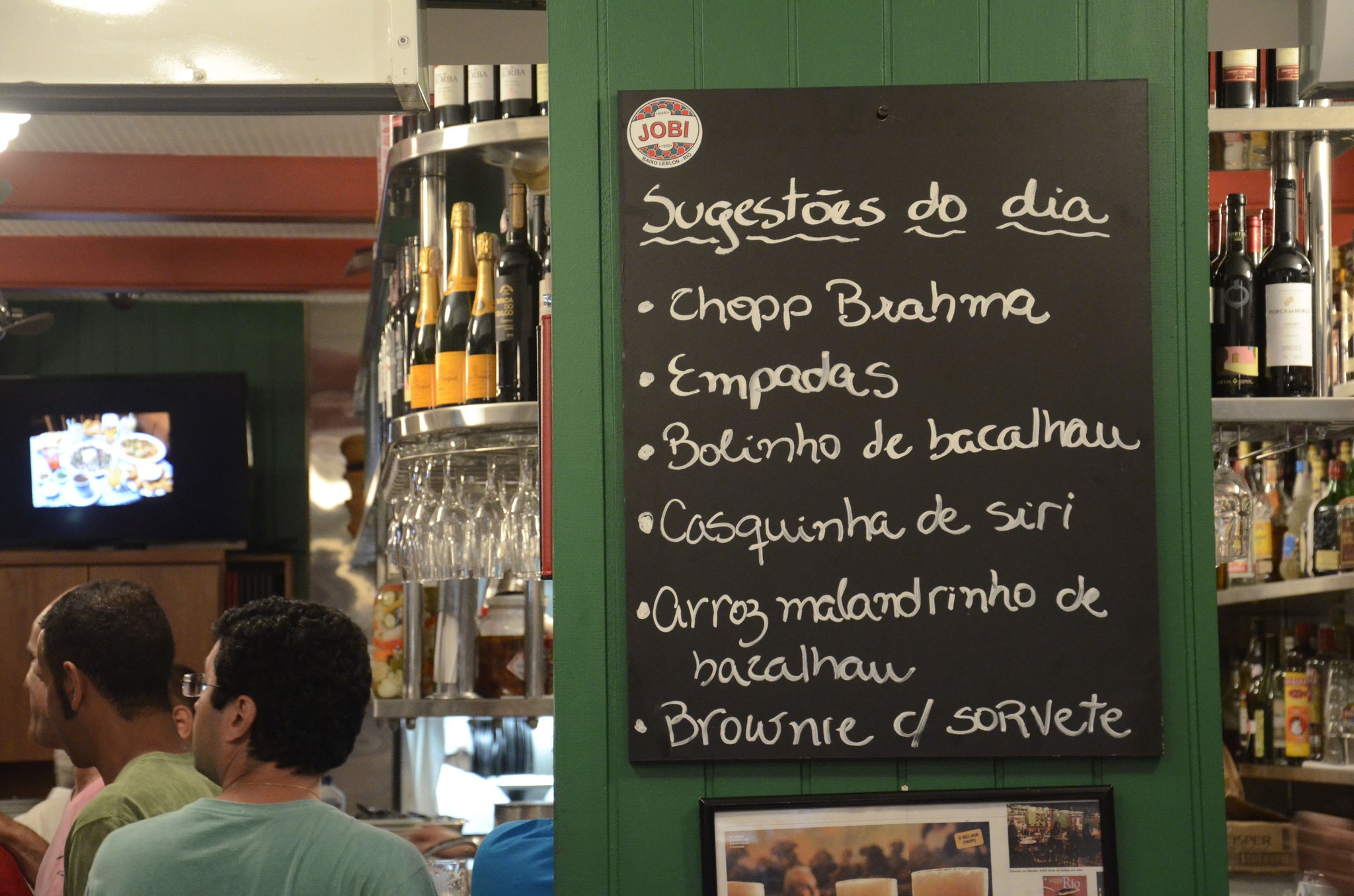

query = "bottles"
372;60;550;230
377;183;553;428
1263;47;1305;107
471;570;555;697
372;580;438;699
1217;590;1353;767
1215;49;1261;108
1209;178;1315;398
1227;423;1353;589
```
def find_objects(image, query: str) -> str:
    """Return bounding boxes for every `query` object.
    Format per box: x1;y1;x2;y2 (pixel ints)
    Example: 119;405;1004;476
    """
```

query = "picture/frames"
699;785;1120;895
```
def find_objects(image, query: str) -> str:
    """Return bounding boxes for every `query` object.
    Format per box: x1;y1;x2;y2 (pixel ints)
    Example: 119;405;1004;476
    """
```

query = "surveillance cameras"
107;290;141;311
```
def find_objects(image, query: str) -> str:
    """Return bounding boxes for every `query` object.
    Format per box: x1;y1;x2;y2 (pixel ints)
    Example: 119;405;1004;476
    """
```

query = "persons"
37;580;221;895
0;587;108;895
80;594;444;895
13;658;512;858
726;803;1100;896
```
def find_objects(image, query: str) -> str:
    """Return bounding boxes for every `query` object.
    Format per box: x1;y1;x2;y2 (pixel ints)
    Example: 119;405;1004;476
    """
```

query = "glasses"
179;671;241;698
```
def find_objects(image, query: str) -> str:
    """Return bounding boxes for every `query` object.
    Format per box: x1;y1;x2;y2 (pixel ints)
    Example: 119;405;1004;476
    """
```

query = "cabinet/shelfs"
350;59;1354;896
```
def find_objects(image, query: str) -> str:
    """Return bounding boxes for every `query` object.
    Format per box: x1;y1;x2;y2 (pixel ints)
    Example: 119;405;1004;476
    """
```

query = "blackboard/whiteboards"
611;75;1164;763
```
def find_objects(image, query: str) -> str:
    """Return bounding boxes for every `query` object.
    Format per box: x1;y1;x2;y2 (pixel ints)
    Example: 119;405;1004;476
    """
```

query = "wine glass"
1213;420;1252;568
383;424;545;584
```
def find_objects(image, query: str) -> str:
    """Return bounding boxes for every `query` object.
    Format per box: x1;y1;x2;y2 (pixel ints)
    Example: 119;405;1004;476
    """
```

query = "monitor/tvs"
2;373;256;554
